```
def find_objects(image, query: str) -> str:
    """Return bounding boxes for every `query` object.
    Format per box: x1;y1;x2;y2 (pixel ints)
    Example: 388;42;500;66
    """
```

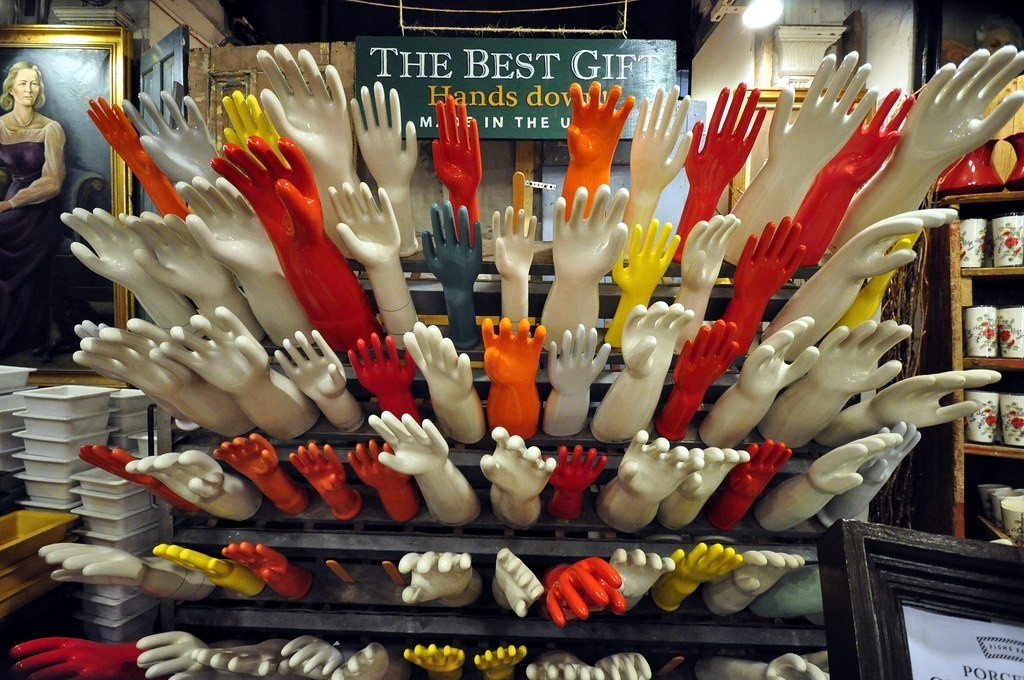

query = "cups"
957;210;1023;270
962;304;1023;358
977;482;1023;541
965;390;1023;446
